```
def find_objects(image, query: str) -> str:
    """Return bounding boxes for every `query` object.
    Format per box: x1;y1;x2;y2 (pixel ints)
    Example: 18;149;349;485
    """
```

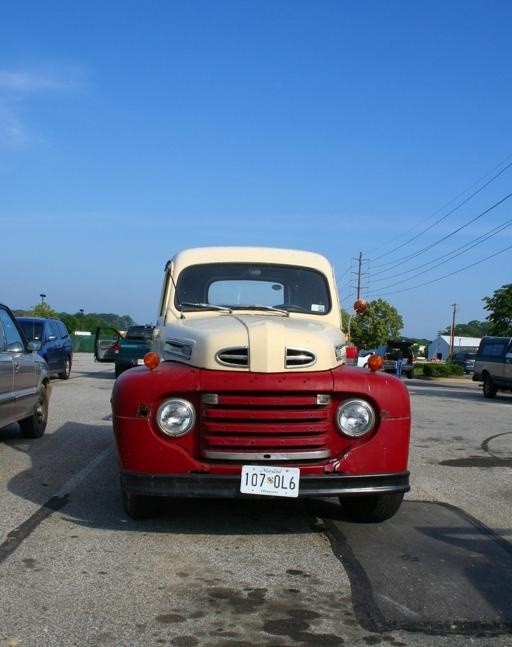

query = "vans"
447;352;477;373
472;336;512;397
0;304;73;437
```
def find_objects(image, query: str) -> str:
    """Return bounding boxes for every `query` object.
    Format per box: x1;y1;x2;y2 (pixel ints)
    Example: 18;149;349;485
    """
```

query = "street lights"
39;293;46;304
79;309;84;329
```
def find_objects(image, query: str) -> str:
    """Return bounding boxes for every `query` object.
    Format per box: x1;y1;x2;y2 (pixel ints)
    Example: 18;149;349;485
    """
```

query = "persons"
397;350;403;378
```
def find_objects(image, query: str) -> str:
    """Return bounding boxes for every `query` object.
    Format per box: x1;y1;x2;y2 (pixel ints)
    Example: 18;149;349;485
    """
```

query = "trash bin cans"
385;354;403;378
